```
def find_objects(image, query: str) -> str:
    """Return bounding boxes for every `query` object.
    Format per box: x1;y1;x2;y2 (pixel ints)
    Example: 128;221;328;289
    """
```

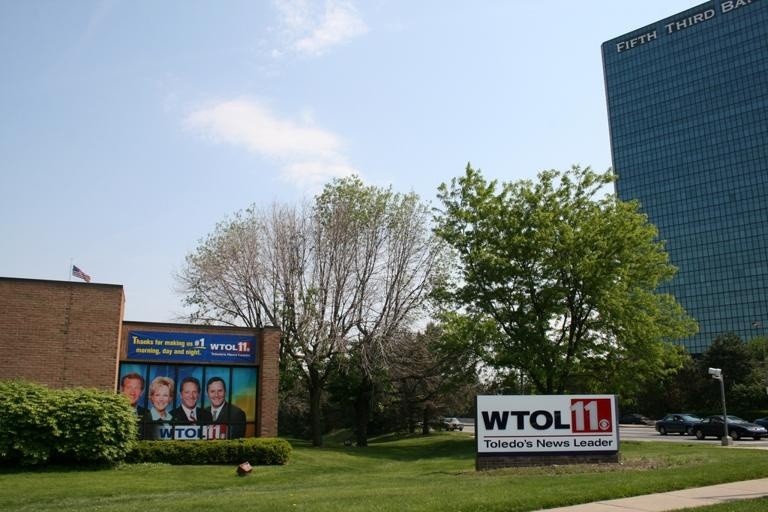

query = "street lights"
708;367;733;445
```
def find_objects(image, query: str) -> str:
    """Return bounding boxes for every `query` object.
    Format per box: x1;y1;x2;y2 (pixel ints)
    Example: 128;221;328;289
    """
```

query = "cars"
444;418;464;431
620;413;649;425
655;414;768;440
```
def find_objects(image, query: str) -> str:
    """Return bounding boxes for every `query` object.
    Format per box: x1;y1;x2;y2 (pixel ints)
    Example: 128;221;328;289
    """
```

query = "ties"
212;409;217;422
190;410;196;423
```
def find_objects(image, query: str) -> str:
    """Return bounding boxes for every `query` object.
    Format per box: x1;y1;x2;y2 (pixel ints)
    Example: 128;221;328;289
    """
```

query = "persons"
121;374;144;423
202;377;246;437
176;376;202;426
146;376;174;425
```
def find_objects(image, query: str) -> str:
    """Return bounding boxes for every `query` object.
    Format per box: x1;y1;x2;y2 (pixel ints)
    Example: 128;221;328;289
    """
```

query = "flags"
72;266;91;282
